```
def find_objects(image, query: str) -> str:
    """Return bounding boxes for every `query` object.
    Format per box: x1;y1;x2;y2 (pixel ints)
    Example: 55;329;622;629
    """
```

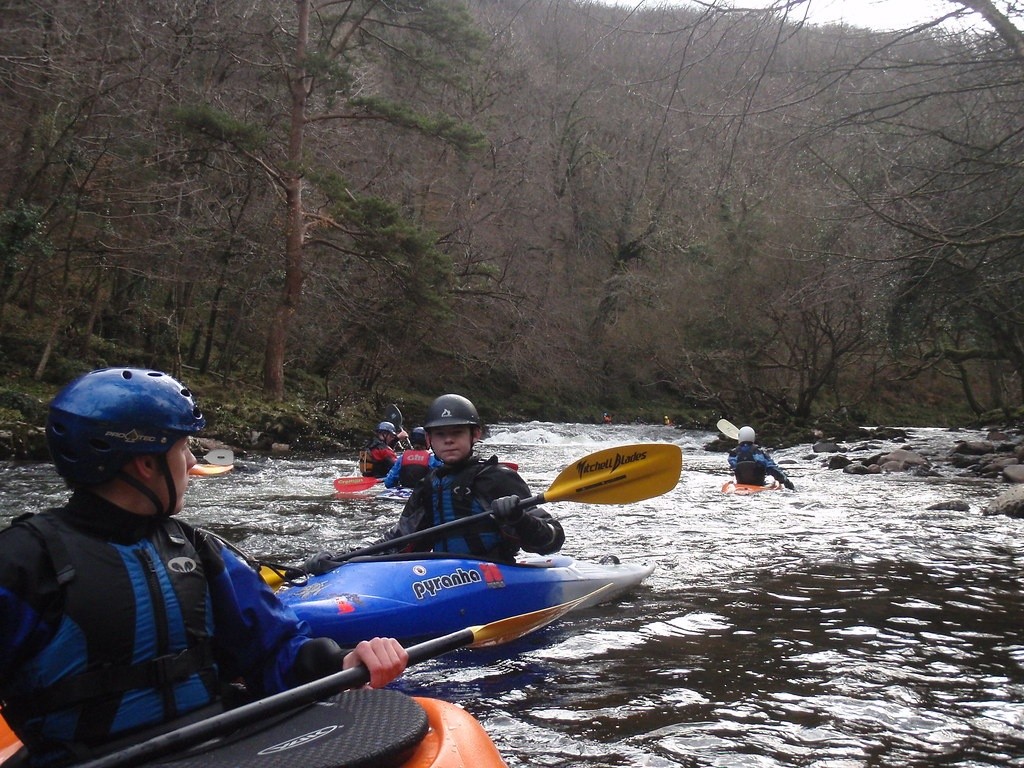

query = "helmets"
375;422;397;436
423;394;480;432
738;425;755;444
409;427;427;446
45;367;206;490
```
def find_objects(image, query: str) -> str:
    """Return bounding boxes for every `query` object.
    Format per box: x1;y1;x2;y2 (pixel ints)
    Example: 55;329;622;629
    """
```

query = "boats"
365;451;520;506
271;545;658;650
720;478;784;496
187;462;235;477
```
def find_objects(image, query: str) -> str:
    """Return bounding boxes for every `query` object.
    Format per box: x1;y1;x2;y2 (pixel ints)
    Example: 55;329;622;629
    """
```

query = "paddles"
194;449;234;465
715;418;741;440
385;405;414;450
71;582;615;768
259;443;683;594
334;463;518;492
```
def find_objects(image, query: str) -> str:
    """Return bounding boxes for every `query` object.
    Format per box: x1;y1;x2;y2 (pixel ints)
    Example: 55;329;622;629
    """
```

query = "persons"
0;367;408;768
728;426;794;490
665;415;670;426
603;412;612;425
358;422;444;488
304;393;564;578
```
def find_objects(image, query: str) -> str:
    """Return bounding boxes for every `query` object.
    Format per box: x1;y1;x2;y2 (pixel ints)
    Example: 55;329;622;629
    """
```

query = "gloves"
784;479;794;490
299;551;334;575
491;495;525;525
